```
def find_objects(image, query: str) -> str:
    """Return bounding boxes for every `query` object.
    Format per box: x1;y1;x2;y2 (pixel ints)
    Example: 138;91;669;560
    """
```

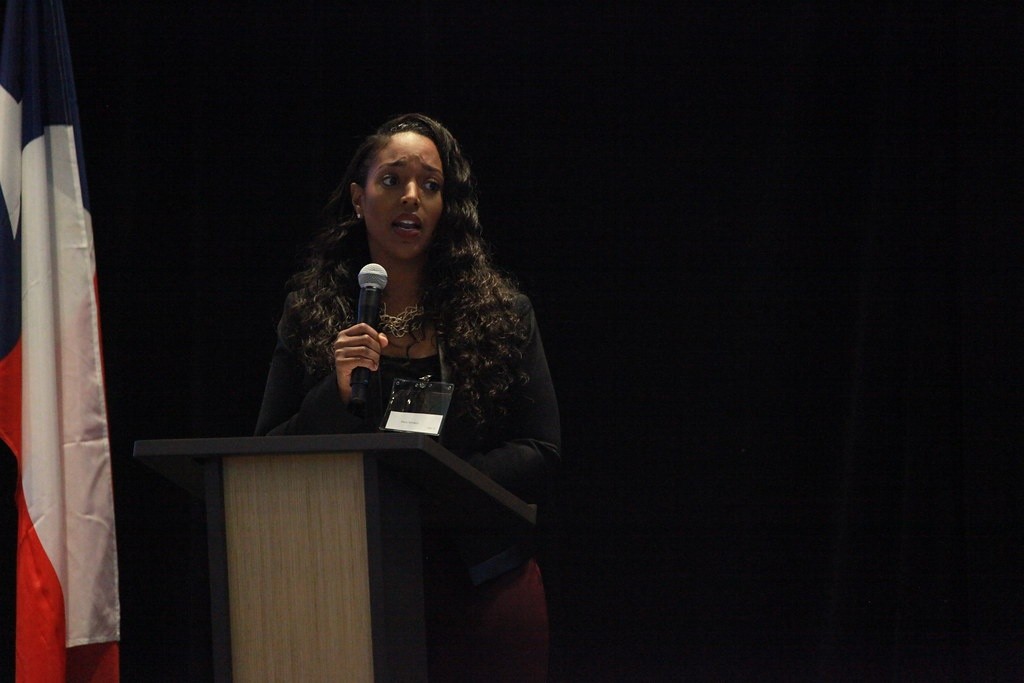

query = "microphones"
349;263;388;403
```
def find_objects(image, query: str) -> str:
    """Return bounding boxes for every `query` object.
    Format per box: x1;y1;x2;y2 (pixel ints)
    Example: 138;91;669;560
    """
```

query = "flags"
0;0;123;682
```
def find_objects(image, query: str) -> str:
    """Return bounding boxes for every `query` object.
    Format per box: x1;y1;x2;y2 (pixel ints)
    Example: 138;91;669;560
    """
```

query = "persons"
254;112;564;683
